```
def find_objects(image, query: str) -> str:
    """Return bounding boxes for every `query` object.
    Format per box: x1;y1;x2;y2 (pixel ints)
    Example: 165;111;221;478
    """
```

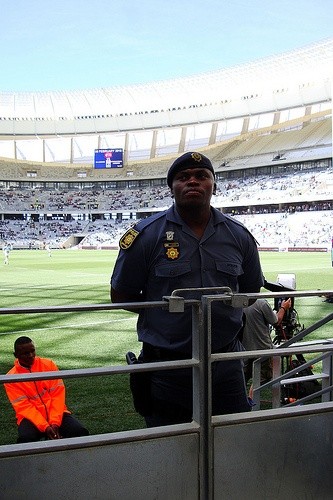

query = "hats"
167;152;214;188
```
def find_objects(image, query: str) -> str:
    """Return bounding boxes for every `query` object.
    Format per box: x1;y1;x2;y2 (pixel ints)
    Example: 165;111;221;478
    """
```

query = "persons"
109;152;263;428
3;337;89;443
0;169;333;250
242;296;292;386
3;244;13;264
45;243;51;257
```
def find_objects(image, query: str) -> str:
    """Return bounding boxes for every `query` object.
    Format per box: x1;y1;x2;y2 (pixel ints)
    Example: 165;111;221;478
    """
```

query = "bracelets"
280;306;286;310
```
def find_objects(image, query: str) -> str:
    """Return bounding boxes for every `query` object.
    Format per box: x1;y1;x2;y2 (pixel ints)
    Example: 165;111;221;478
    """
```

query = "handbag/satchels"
130;354;177;416
